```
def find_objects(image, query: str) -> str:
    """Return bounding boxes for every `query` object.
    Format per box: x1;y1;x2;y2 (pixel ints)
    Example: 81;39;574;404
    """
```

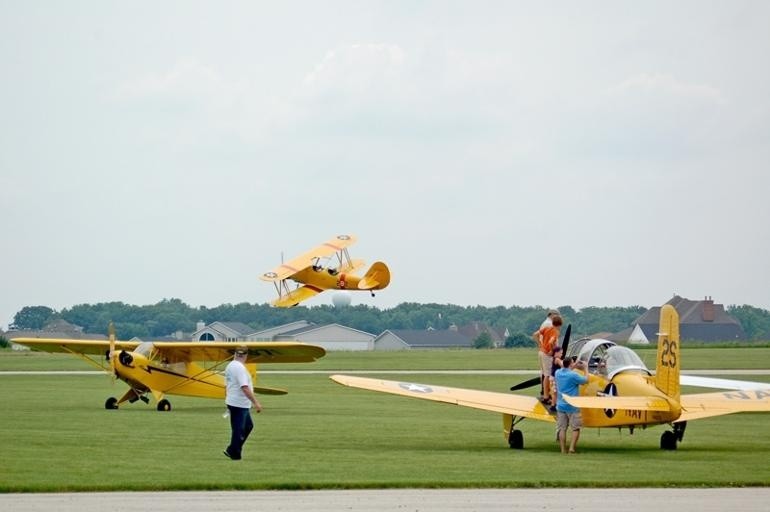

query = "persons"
548;346;563;411
554;356;590;454
537;308;560;400
532;315;562;405
222;344;261;459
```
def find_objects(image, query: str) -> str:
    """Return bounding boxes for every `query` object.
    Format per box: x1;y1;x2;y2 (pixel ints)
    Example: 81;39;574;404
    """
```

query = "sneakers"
541;398;552;405
550;405;557;412
223;449;231;458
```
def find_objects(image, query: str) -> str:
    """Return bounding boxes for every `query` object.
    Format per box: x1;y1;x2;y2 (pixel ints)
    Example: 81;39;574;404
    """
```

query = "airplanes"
259;234;390;309
9;322;326;411
330;304;770;449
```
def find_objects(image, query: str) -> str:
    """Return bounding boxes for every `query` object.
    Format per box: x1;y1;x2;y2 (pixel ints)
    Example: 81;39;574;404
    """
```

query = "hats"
235;346;249;355
